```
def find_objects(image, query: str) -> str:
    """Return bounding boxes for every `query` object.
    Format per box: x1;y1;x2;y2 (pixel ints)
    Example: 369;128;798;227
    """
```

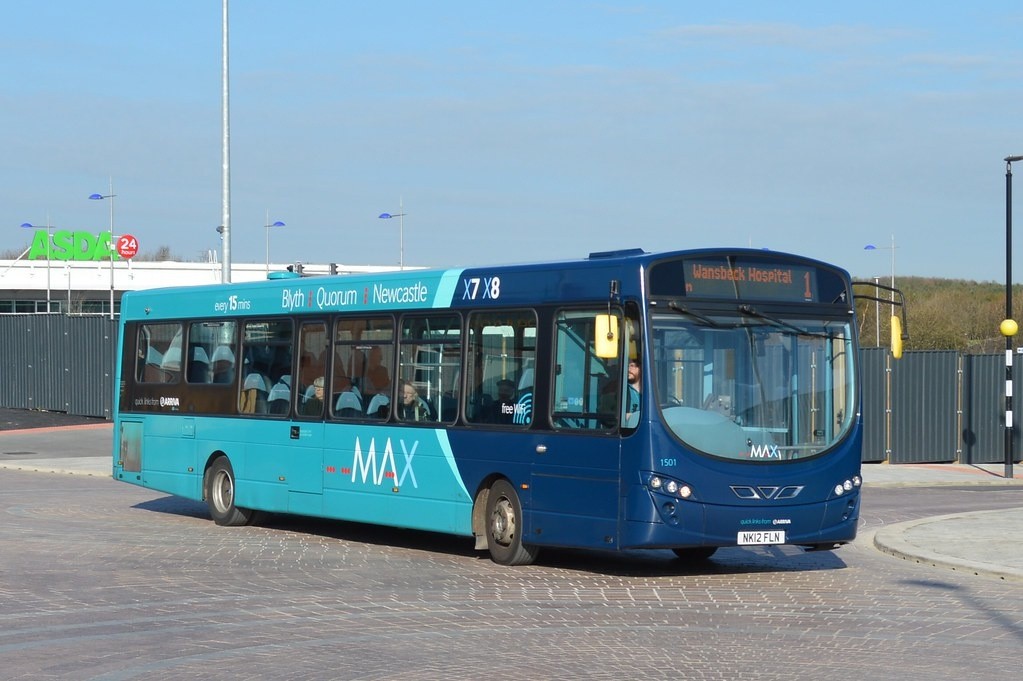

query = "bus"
113;248;908;566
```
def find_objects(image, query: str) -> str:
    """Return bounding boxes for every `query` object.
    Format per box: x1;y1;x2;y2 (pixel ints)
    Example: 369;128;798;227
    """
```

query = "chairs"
141;341;535;421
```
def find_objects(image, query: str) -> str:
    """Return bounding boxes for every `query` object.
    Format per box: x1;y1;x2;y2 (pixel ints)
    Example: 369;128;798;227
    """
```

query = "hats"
314;376;324;388
496;379;515;387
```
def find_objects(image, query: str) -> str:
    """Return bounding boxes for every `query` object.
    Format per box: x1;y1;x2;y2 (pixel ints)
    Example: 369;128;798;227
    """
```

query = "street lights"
20;213;55;312
88;176;118;319
264;210;287;275
378;197;407;270
1001;154;1023;478
864;234;897;350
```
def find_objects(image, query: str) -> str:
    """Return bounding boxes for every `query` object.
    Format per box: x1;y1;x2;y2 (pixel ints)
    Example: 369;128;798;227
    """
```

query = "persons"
269;331;388;394
480;379;518;423
387;379;433;422
600;352;640;421
303;376;334;416
